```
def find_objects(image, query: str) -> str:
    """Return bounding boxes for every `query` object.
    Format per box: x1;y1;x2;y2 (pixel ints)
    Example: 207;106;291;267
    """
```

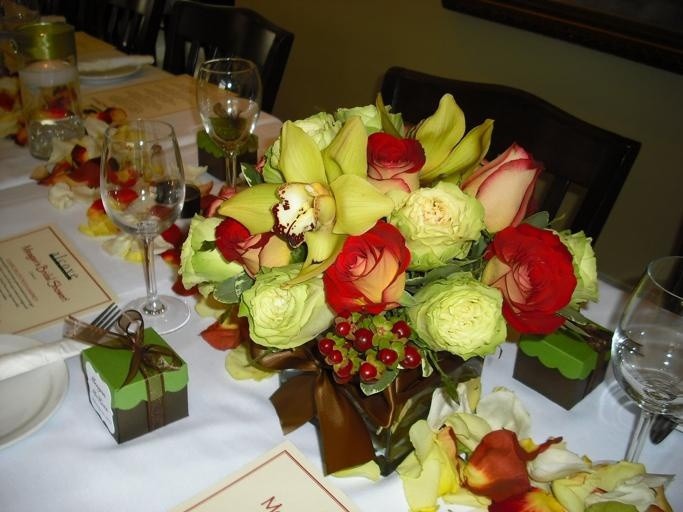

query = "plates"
79;65;142;81
0;333;70;454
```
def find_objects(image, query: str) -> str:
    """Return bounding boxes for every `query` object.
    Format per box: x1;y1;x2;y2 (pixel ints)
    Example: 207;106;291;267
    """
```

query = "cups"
4;0;40;31
17;58;81;160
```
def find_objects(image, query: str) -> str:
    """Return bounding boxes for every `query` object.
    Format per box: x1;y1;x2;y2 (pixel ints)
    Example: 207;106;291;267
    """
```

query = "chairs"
18;1;294;114
381;65;643;249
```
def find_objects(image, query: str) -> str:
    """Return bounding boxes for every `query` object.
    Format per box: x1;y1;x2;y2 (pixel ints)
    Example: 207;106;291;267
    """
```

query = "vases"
279;329;483;475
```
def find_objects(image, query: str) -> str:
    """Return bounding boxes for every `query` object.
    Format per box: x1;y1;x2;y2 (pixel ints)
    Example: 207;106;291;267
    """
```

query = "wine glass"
194;58;263;195
99;120;191;337
612;255;681;469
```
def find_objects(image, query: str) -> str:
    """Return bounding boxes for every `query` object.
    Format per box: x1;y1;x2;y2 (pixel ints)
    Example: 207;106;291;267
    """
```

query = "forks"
89;302;122;332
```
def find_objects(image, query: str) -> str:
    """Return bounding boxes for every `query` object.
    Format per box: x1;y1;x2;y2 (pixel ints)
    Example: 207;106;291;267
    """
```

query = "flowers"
178;91;600;396
